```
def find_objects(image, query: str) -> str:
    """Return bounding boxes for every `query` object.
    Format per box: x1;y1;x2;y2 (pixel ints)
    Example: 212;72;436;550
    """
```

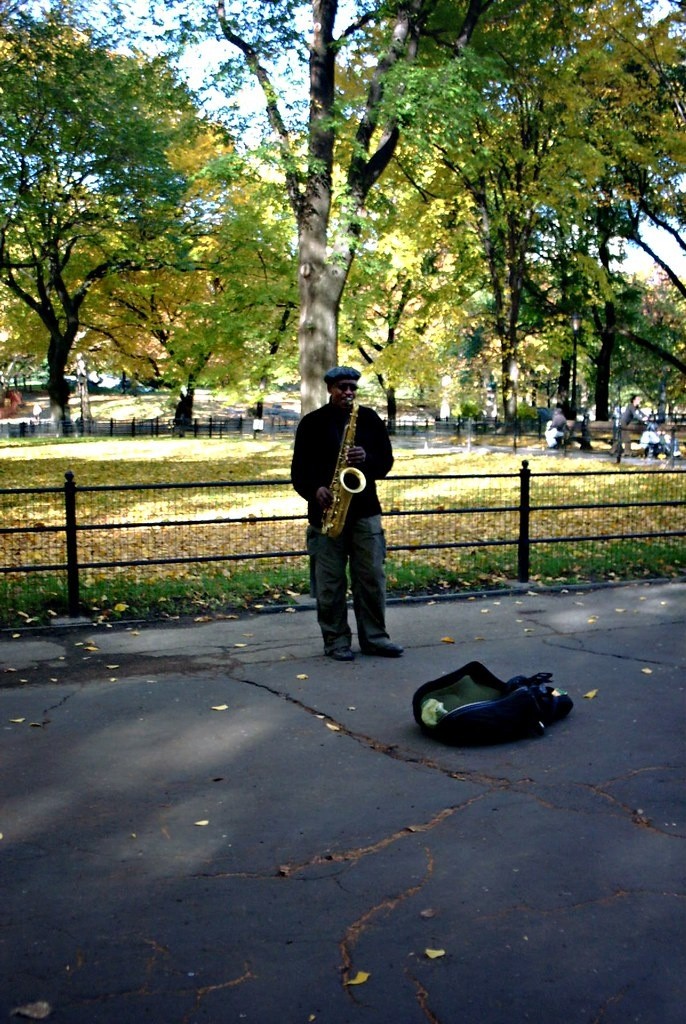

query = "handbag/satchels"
413;662;572;748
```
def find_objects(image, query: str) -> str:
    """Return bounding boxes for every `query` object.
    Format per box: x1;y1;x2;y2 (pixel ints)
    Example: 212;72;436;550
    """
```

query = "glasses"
330;383;359;392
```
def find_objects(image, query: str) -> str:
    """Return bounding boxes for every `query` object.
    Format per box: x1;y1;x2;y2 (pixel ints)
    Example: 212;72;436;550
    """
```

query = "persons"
606;395;650;457
545;408;567;447
291;366;404;661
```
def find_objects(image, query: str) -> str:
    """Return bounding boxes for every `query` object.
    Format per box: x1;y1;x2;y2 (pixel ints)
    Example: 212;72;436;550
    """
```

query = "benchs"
567;420;686;458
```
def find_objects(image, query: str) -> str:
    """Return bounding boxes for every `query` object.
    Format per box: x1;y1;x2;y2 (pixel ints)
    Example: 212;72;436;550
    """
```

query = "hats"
324;366;361;384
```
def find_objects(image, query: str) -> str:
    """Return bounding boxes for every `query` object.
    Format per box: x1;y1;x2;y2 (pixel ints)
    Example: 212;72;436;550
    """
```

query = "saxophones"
319;396;367;538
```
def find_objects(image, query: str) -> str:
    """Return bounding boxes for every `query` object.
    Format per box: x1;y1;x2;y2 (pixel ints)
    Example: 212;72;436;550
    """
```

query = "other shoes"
374;646;404;657
333;647;354;661
